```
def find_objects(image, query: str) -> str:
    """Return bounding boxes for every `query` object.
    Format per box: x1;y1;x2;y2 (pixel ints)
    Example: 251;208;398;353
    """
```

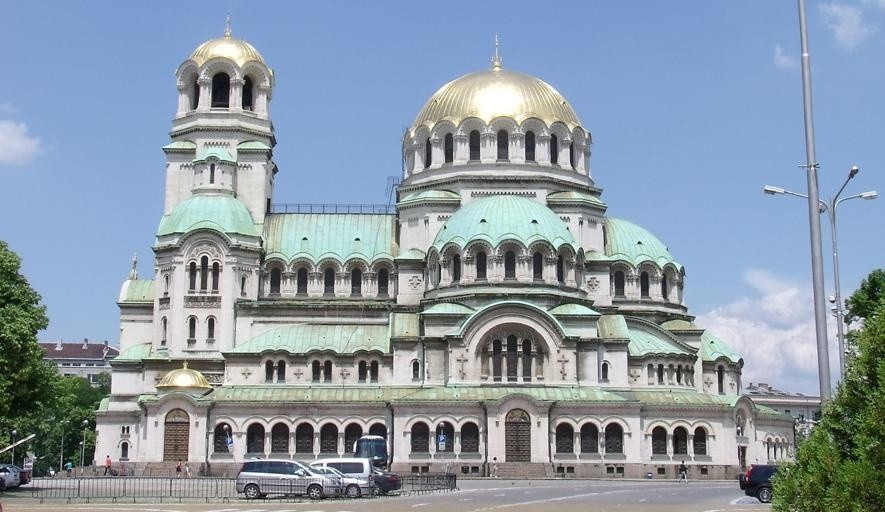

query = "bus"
353;436;390;471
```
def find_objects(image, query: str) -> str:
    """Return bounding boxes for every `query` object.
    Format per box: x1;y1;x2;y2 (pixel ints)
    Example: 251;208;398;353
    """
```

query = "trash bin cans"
648;473;652;479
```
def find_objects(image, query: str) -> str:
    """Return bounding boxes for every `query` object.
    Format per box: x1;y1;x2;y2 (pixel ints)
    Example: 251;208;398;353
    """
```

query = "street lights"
79;441;83;465
736;426;742;466
440;422;444;459
11;430;17;464
763;166;880;400
81;420;89;476
58;416;70;472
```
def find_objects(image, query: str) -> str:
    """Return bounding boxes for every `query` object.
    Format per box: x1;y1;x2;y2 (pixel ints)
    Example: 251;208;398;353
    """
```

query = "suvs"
737;464;796;503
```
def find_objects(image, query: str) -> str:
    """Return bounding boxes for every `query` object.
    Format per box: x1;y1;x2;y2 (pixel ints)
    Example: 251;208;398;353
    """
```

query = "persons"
104;454;112;475
677;460;690;484
175;455;182;478
184;459;192;479
63;460;73;479
490;456;499;479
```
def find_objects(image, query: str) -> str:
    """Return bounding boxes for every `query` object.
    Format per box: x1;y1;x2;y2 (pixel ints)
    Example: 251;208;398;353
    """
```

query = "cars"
293;466;369;499
9;464;31;485
0;465;21;488
0;478;6;492
372;467;402;496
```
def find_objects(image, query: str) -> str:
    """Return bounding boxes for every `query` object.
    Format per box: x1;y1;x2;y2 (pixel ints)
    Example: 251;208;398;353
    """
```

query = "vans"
294;457;374;498
235;458;342;500
